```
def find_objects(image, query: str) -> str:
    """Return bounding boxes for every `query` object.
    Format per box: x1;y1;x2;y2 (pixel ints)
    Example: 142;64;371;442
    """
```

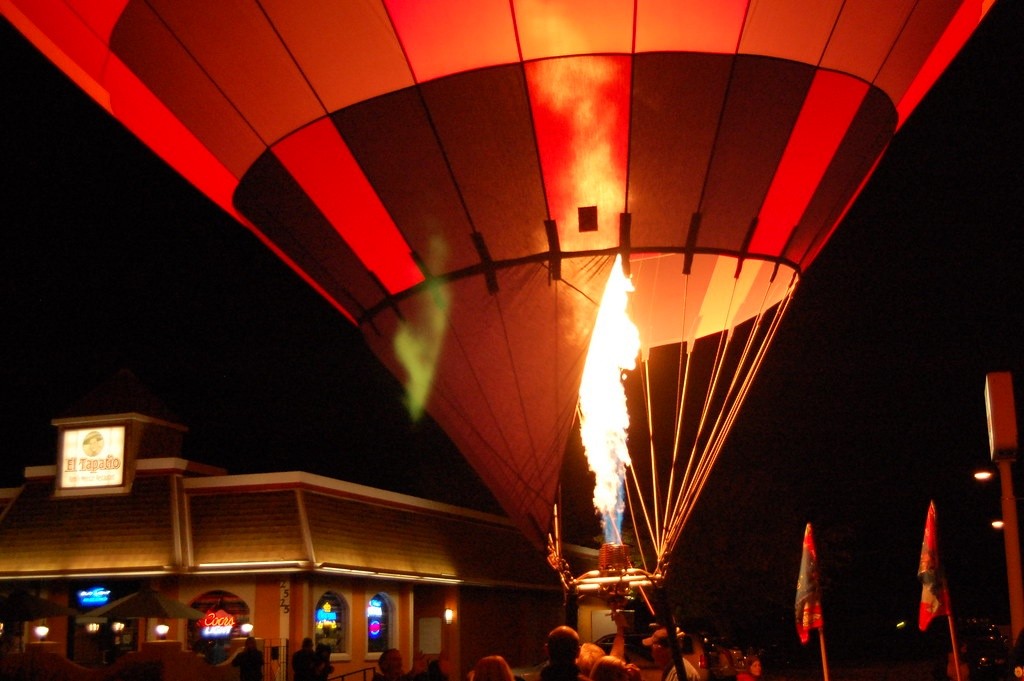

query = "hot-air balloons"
0;0;998;617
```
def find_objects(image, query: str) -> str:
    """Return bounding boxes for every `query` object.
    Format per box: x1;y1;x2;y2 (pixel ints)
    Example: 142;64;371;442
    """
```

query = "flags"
918;500;950;630
793;521;825;645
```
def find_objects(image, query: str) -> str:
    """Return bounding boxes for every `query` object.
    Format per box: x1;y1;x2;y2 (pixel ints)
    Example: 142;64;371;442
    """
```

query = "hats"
642;629;667;645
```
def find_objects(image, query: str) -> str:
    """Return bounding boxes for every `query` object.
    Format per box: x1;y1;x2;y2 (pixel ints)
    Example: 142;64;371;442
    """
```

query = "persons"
232;636;263;681
292;638;334;681
371;624;763;681
946;639;973;681
